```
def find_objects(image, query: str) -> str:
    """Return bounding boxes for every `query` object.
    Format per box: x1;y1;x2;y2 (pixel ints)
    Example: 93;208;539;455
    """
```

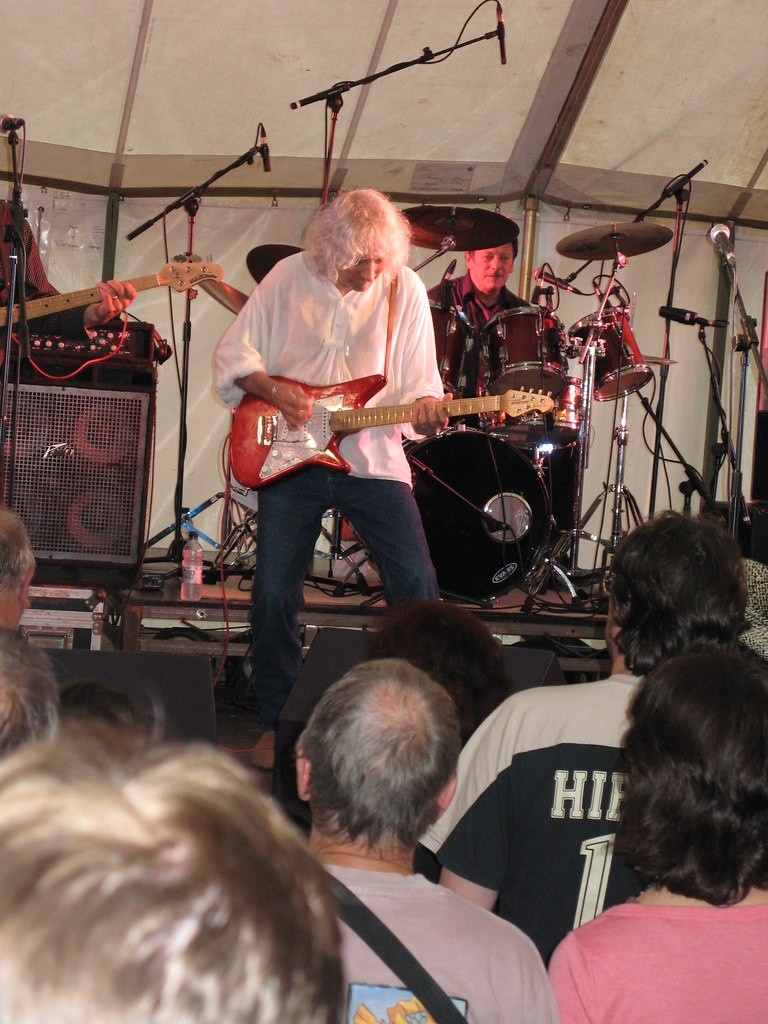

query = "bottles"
181;531;203;601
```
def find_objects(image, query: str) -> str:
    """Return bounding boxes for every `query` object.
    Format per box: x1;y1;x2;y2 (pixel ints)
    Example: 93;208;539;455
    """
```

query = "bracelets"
271;381;284;413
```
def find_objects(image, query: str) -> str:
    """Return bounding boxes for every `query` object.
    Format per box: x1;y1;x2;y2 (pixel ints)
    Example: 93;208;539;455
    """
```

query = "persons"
428;235;570;603
0;509;768;1024
0;198;137;363
216;187;454;770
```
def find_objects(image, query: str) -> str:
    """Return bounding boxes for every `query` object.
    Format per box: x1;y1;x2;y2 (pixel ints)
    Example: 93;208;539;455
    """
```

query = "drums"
568;306;654;402
428;298;479;400
480;376;584;448
479;306;568;401
400;427;550;603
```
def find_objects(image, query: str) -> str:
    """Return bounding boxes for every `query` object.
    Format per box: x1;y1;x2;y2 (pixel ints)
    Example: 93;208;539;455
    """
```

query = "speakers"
46;648;215;745
274;627;568;805
0;354;158;572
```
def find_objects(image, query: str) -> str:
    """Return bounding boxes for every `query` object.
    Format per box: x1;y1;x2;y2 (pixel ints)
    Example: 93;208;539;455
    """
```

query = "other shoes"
250;730;274;771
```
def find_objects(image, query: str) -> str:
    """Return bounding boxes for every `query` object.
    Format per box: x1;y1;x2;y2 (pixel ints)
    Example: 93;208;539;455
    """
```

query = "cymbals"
246;243;307;285
400;205;521;251
555;221;674;260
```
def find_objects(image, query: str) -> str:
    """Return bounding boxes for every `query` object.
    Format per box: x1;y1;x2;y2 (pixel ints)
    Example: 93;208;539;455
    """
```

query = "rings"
112;295;117;300
421;424;430;430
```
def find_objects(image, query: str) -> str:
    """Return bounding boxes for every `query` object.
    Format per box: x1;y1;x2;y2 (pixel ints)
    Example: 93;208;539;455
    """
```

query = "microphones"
659;306;729;327
260;125;271;172
532;268;581;295
593;281;612;308
531;269;545;305
496;3;506;65
0;113;25;133
488;520;512;532
711;224;736;269
436;262;456;296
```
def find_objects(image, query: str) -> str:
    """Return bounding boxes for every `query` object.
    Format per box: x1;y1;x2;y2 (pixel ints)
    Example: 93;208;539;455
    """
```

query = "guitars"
229;374;555;490
0;252;225;329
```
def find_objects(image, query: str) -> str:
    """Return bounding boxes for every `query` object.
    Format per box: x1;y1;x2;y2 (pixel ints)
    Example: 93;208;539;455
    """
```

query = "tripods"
127;147;649;612
